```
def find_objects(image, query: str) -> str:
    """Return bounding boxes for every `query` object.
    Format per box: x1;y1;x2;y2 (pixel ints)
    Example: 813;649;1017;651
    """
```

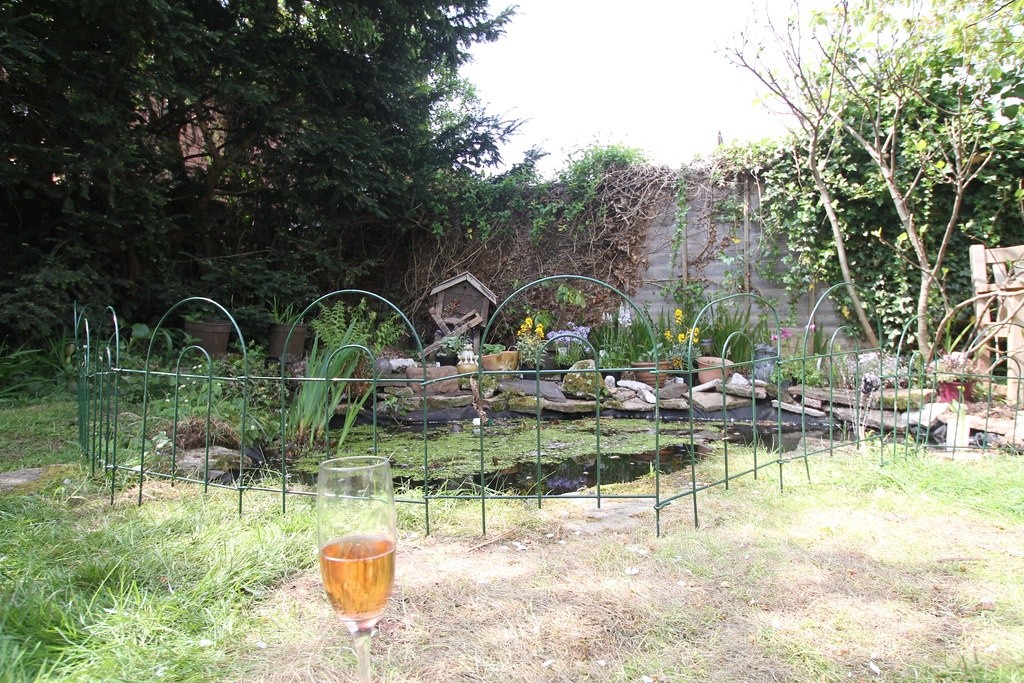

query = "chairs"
969;244;1024;405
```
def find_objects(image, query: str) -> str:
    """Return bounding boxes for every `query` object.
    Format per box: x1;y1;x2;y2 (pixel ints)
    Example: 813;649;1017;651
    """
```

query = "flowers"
515;318;547;367
547;321;589;365
772;323;827;384
926;351;983;385
665;309;702;370
598;301;632;369
852;353;911;388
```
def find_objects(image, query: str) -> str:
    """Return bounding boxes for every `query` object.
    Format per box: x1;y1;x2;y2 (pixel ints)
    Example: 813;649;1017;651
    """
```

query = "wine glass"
317;456;396;683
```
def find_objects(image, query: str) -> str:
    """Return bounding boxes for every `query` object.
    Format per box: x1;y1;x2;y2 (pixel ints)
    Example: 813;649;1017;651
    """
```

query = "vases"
671;364;698;387
558;363;573;381
600;367;623;388
940;381;972;402
520;363;543;379
696;357;736;385
184;317;231;357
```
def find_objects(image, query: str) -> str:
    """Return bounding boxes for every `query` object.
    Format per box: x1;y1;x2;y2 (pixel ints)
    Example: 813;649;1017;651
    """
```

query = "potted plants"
481;344;519;370
270;306;309;361
631;306;671;388
711;308;767;380
